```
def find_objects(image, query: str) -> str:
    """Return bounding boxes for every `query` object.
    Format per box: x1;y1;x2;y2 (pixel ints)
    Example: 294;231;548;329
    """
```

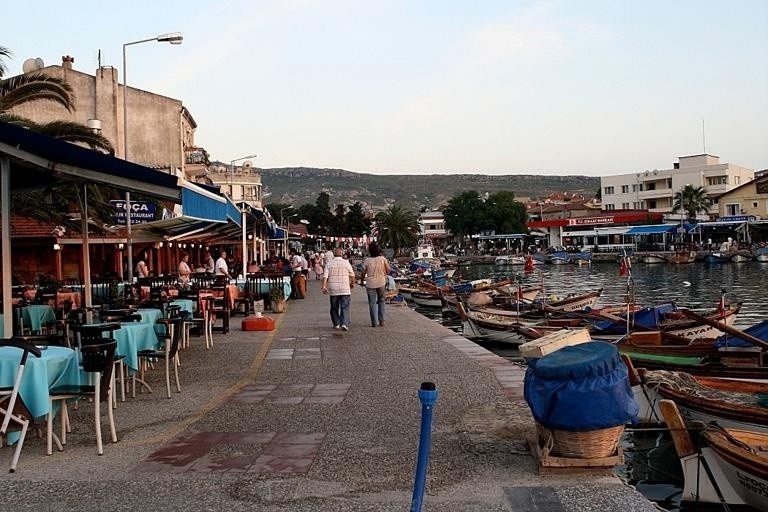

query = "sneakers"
342;324;348;331
334;325;340;329
373;322;384;327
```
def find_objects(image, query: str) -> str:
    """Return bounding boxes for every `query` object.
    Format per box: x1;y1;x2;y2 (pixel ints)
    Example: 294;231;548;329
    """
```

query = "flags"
618;259;627;276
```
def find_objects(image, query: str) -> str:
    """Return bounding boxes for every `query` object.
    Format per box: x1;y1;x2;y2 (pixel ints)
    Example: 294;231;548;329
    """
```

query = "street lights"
124;32;184;283
636;169;659;210
231;154;257;182
259;192;298;238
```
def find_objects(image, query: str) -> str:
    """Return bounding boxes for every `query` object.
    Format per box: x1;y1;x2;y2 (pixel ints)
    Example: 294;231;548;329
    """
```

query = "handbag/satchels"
386;276;395;291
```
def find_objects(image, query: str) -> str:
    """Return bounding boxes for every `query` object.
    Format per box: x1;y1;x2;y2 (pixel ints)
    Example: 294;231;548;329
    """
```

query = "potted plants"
269;281;290;314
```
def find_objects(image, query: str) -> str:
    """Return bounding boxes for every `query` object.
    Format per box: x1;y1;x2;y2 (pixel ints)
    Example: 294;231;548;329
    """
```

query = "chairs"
112;354;127;408
1;272;252;349
136;316;185;399
0;338;42;471
46;337;118;456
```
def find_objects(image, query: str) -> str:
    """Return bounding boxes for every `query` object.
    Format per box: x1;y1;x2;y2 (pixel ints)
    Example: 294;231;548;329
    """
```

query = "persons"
177;250;194;285
203;250;216;274
321;247;356;331
134;249;150;279
247;261;260;273
214;249;233;280
360;244;391;327
707;236;713;253
278;242;362;300
